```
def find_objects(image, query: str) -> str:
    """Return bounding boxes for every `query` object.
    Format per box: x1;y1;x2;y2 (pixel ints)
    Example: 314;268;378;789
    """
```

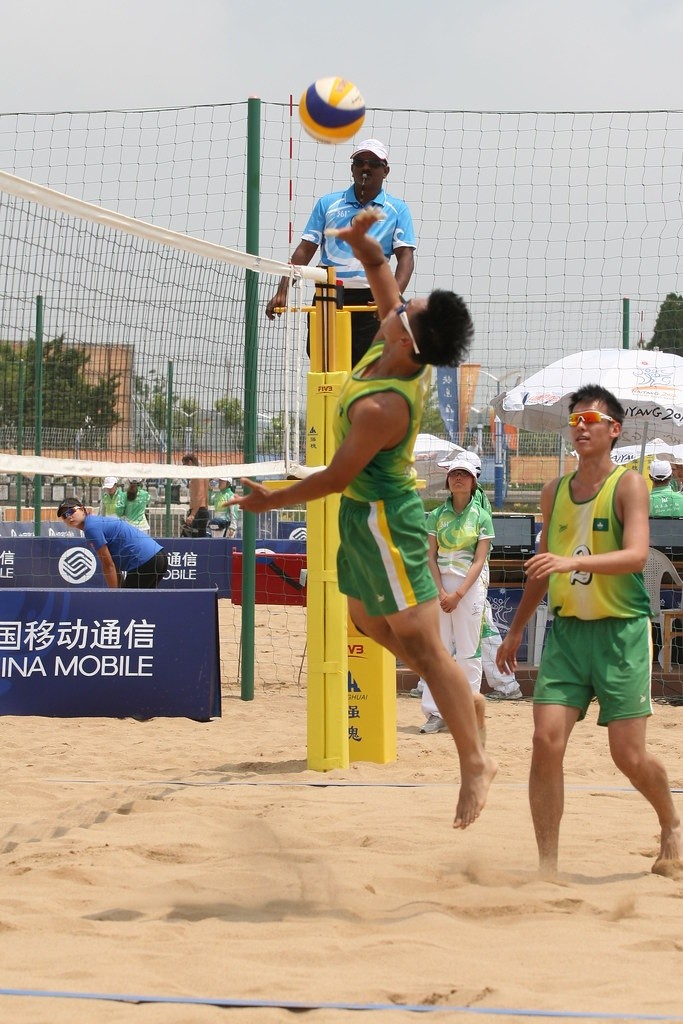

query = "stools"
662;611;683;673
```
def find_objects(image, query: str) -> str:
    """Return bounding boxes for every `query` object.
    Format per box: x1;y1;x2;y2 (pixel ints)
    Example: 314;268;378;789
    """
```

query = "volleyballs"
299;78;366;144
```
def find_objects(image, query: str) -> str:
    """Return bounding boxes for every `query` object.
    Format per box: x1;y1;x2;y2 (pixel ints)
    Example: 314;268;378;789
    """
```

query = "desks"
486;560;683;666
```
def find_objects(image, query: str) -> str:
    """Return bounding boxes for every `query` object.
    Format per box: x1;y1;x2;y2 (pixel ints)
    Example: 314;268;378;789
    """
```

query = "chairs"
642;547;683;672
527;530;555;668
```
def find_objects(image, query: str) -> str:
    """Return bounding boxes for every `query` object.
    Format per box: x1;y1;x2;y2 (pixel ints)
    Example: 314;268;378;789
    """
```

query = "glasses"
397;300;420;356
568;411;621;427
352;157;386;169
448;470;470;477
61;505;81;519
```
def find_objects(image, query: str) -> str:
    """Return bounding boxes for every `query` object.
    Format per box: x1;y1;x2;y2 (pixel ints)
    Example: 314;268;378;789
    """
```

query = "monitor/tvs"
649;515;683;562
489;514;536;561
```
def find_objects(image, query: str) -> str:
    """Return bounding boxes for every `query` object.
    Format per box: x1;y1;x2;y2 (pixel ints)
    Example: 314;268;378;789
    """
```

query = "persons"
99;477;122;517
648;459;683;517
221;205;500;829
266;136;416;371
180;454;209;538
467;437;483;455
420;451;522;733
496;385;683;879
208;478;238;537
57;498;168;589
115;477;151;531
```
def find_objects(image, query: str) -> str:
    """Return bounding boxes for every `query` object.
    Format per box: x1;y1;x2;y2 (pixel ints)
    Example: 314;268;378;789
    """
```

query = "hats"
437;450;481;474
57;498;81;517
649;460;672;481
101;477;118;489
129;477;143;484
350;139;388;166
445;460;477;486
215;477;232;487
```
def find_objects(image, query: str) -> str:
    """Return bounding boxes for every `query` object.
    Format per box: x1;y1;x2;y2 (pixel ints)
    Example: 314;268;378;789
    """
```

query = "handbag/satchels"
208;517;230;530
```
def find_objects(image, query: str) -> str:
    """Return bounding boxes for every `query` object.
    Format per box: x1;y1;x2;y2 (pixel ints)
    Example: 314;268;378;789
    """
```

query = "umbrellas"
492;348;683;464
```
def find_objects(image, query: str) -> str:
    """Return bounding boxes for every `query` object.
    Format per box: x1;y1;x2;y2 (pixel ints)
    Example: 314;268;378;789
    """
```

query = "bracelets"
456;591;462;599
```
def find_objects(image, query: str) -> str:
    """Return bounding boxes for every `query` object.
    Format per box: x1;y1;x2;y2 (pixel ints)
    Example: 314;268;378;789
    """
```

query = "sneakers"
410;685;423;697
484;686;523;701
419;715;449;734
